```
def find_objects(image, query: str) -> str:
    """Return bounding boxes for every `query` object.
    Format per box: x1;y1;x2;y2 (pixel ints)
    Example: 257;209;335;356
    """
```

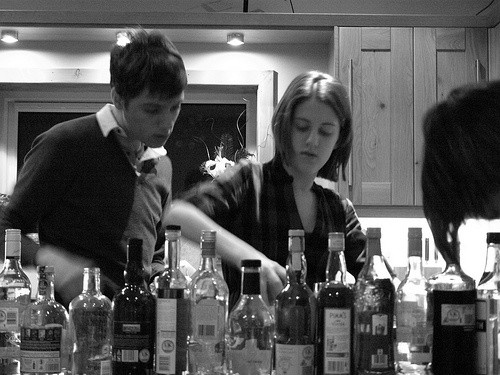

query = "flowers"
192;98;272;182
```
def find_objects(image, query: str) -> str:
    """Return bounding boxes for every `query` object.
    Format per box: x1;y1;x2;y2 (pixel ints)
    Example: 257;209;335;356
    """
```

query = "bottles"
151;224;188;374
68;268;113;375
272;228;316;375
225;259;274;375
314;231;353;375
352;228;394;374
189;229;230;375
392;227;432;374
0;228;32;375
475;232;500;375
425;240;478;374
19;266;69;375
110;237;155;375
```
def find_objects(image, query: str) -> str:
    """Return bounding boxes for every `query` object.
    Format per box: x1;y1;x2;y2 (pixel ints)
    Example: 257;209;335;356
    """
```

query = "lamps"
116;32;131;47
1;31;18;43
227;34;244;45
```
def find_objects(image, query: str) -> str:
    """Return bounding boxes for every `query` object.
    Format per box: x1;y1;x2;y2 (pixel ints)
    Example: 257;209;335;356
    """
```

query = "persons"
163;70;399;305
420;80;500;271
0;28;192;313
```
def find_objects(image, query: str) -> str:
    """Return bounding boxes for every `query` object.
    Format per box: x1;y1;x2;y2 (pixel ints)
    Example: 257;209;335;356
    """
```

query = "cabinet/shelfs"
328;26;488;206
